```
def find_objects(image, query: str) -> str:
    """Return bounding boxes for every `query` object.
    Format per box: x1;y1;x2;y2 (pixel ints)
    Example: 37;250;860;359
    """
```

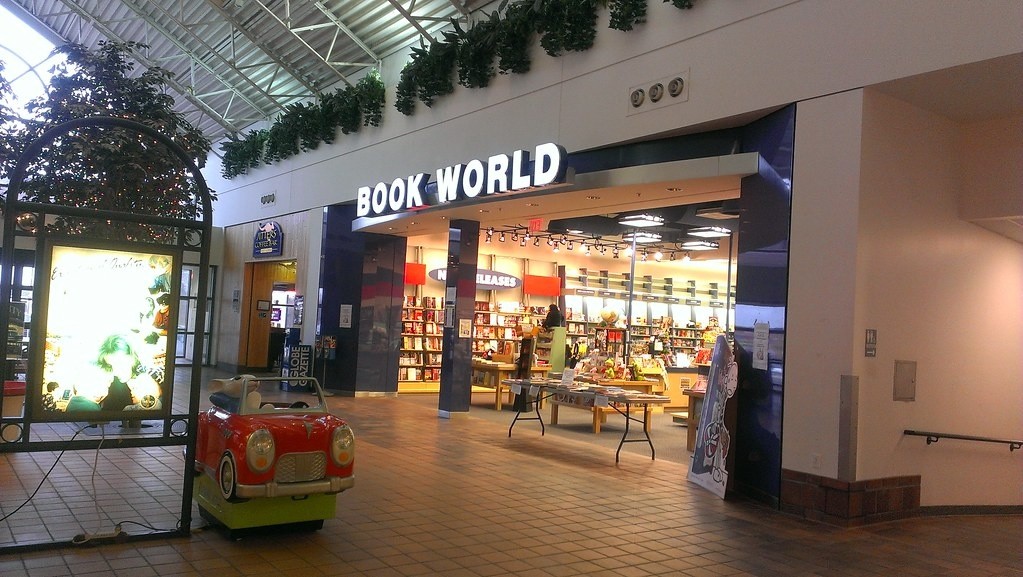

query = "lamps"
485;227;691;262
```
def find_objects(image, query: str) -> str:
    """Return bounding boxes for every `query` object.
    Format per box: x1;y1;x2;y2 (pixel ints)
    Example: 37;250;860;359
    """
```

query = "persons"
541;304;566;328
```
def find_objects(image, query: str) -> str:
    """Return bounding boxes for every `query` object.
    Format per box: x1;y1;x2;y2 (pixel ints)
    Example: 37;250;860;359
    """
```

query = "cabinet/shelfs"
398;305;734;383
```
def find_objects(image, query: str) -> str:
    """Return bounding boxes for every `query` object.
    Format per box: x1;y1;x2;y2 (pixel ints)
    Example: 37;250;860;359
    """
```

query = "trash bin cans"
281;328;311;392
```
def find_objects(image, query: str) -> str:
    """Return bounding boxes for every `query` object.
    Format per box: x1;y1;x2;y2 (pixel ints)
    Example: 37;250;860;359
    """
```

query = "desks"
470;359;711;464
